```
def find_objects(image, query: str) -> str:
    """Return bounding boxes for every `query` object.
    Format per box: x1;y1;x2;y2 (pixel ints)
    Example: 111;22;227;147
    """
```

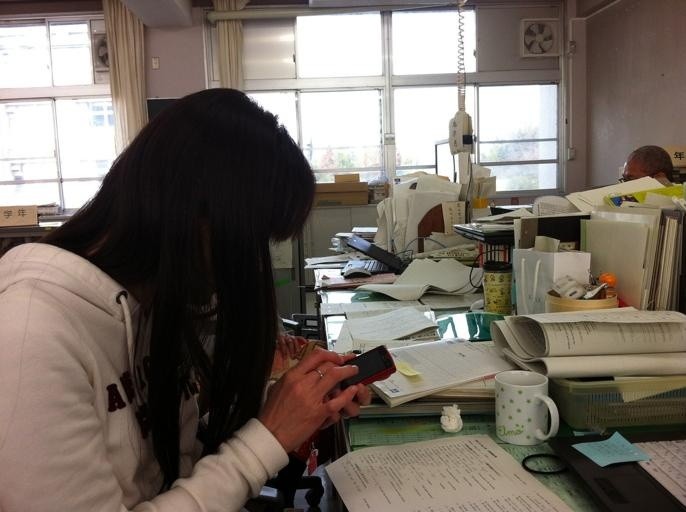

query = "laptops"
548;431;686;512
341;235;405;275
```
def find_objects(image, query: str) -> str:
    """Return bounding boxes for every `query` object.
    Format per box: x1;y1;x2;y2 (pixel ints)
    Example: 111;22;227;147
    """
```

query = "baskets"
554;378;686;430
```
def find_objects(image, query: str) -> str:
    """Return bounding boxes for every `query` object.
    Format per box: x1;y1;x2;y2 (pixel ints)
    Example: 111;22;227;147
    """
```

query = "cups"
494;370;561;449
481;259;515;315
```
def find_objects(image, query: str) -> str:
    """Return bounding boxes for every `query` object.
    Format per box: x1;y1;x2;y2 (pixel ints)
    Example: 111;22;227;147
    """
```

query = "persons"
275;332;309;360
37;204;62;215
0;86;378;511
621;145;675;187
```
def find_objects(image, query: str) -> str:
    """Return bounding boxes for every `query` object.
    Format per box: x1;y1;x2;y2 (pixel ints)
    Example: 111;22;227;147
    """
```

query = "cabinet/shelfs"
303;204;377;288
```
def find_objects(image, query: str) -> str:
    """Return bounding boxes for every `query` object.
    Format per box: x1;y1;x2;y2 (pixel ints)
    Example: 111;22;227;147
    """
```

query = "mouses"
343;268;371;279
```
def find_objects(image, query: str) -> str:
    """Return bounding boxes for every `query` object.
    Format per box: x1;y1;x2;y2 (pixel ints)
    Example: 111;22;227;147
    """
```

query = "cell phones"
336;345;396;399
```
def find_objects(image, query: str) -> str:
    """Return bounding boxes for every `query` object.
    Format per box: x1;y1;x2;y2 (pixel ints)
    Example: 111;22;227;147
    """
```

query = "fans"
91;34;109;72
520;18;562;58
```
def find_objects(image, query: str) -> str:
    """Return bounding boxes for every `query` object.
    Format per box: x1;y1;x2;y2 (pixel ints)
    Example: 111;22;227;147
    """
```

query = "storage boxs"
312;182;368;207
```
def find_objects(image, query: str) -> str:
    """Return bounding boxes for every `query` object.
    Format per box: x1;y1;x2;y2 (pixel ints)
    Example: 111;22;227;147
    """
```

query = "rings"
314;368;324;378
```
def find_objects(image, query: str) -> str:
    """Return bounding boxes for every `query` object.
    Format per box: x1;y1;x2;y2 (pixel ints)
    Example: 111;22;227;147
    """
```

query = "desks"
0;218;65;255
312;252;600;508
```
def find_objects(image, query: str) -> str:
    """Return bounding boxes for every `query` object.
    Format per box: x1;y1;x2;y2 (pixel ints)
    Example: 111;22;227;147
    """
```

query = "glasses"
618;173;657;183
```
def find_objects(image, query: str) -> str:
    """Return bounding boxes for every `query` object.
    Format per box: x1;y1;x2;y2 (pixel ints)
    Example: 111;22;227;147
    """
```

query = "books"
332;171;686;419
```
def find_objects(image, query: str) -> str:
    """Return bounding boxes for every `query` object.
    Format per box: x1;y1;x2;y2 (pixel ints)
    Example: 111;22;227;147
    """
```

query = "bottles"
598;273;617;299
